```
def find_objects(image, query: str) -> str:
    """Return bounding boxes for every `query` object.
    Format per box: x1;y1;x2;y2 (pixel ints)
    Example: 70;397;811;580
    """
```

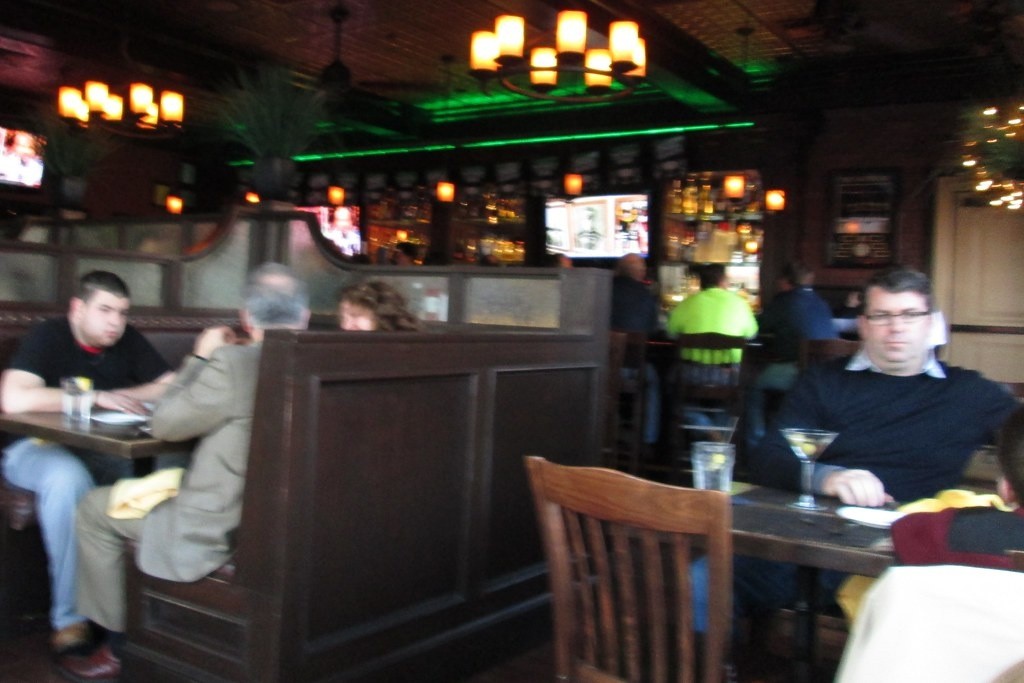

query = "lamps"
469;10;648;103
55;80;187;139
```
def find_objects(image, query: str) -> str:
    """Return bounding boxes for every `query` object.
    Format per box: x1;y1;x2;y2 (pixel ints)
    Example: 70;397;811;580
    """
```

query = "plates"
835;506;897;529
89;413;151;424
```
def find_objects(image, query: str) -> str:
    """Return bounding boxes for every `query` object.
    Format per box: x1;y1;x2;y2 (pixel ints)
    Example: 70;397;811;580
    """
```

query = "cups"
66;375;92;424
692;441;735;494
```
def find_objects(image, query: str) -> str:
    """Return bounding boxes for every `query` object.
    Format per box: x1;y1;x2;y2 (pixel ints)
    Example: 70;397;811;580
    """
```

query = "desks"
605;468;996;683
0;412;198;480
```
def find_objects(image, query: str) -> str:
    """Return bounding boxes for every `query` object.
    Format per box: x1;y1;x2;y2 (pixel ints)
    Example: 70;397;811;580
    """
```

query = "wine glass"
779;428;839;511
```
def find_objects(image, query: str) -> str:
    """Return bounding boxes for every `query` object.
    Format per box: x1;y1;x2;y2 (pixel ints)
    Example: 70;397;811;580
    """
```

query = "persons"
336;279;424;330
322;206;363;259
747;265;838;445
834;407;1024;683
839;279;875;342
394;243;420;266
544;251;571;268
439;247;464;265
481;255;500;266
65;263;315;677
609;252;661;445
683;264;1024;683
665;265;759;442
0;268;168;683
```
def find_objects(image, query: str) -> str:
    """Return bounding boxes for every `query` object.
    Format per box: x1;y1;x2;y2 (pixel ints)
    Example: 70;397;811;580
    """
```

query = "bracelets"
189;352;208;362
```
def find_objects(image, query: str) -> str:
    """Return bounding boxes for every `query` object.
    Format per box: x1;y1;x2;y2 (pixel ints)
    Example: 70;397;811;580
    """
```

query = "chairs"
609;327;863;477
523;455;733;683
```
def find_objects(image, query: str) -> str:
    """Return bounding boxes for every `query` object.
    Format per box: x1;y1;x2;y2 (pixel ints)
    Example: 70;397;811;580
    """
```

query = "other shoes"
50;638;101;669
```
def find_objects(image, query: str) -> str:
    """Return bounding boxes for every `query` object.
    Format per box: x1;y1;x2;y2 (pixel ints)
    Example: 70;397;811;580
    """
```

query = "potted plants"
215;47;346;202
37;118;120;213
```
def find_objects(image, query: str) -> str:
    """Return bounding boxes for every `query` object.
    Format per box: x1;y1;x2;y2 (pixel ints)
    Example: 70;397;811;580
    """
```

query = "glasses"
860;311;929;326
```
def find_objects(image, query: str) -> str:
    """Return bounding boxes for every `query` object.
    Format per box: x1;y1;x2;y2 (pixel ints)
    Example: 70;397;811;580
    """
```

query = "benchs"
120;325;596;683
0;308;248;620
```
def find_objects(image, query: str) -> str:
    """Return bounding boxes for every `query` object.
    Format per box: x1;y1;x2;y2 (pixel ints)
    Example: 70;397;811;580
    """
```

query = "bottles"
370;190;525;261
658;176;764;315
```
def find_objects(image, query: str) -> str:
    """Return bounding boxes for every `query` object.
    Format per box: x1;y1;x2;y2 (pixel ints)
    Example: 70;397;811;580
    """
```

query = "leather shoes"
56;641;122;683
